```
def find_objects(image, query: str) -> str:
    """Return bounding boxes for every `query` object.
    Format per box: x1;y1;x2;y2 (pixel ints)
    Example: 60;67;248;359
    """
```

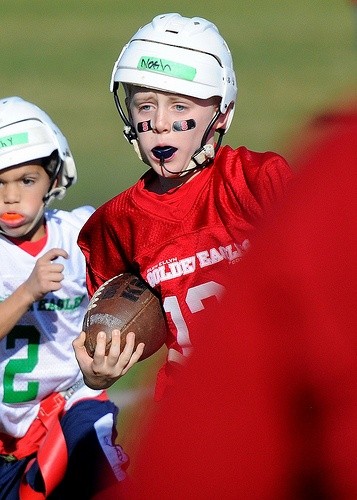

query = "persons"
1;96;130;500
73;12;292;406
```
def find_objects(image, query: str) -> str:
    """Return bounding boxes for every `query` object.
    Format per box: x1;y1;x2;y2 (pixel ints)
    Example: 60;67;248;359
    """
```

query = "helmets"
0;97;77;237
109;13;237;179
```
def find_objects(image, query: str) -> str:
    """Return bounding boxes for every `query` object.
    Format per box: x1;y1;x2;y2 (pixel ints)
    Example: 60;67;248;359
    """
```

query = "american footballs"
82;273;165;364
